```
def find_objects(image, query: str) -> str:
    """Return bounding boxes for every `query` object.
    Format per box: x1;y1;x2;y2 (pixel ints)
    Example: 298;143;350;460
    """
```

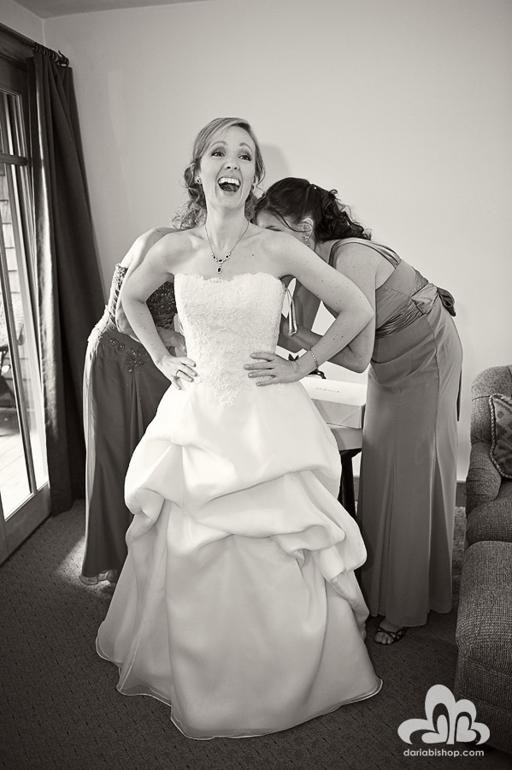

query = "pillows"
486;392;511;481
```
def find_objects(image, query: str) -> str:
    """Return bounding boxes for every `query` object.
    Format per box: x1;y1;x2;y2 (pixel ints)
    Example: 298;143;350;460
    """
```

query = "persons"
78;194;261;586
261;178;463;646
93;115;383;739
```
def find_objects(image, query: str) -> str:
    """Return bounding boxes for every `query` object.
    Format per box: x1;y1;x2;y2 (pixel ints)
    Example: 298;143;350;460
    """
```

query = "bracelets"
309;349;320;368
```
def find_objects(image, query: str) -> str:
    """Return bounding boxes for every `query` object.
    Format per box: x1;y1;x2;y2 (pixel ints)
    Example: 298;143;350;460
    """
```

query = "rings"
175;370;180;376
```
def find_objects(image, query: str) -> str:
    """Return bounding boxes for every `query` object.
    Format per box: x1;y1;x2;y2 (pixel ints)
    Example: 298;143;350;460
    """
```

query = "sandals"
374;616;406;646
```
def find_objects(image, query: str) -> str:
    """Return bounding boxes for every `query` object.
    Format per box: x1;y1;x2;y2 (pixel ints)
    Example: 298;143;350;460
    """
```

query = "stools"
454;541;510;755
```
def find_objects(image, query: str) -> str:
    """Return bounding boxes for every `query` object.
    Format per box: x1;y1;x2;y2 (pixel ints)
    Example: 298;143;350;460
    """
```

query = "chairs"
468;363;511;541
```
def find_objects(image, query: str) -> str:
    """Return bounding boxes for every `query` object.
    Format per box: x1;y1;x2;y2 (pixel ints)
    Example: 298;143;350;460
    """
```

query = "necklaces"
204;221;250;273
